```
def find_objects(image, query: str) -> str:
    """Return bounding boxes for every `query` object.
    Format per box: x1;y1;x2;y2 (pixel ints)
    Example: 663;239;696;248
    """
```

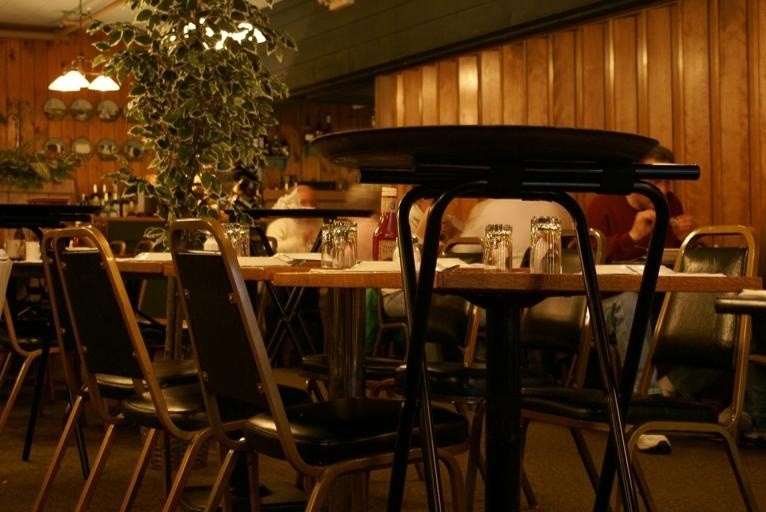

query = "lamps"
45;1;121;96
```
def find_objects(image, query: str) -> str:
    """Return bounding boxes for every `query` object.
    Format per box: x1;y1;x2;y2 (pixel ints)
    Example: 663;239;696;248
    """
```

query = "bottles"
371;185;417;269
268;176;299;189
245;125;291;157
304;112;334;144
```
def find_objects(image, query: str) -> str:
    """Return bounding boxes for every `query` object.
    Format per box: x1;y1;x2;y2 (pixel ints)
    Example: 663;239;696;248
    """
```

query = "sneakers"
636;433;674;456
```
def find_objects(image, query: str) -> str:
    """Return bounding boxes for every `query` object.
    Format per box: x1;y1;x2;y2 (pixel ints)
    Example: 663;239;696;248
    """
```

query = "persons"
583;140;714;455
262;178;332;327
380;185;472;392
463;187;576;313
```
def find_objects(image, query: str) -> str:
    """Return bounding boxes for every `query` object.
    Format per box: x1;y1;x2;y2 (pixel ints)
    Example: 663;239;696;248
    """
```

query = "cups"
78;181;136;217
219;222;254;258
482;221;516;272
6;238;42;261
321;218;359;271
530;215;561;273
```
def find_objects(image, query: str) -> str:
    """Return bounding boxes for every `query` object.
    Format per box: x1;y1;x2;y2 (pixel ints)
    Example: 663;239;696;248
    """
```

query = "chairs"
0;300;87;428
163;219;469;511
31;225;260;511
302;228;756;511
